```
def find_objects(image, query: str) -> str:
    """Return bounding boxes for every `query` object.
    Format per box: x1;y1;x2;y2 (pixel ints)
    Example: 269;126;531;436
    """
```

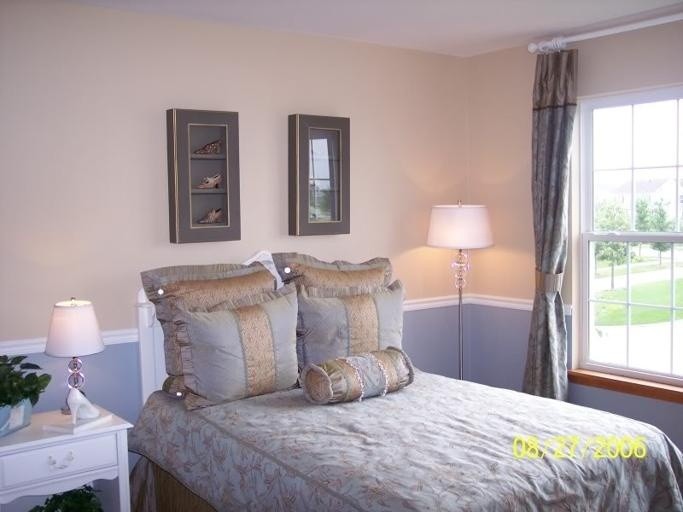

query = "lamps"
133;248;683;512
423;198;495;380
43;297;107;415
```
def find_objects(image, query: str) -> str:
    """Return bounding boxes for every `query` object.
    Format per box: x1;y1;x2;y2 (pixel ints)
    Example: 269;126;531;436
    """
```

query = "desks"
0;403;135;511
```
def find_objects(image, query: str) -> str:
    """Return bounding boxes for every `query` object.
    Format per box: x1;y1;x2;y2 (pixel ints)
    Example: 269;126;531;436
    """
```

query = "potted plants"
1;354;52;440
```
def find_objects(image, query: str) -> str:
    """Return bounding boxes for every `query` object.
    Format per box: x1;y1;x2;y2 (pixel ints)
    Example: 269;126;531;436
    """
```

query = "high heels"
194;138;223;154
198;207;223;224
66;387;101;425
196;172;223;189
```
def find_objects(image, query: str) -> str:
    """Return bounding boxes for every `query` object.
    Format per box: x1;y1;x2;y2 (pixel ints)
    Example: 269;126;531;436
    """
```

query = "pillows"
271;251;394;285
140;253;277;397
293;281;404;376
299;346;414;405
165;284;298;411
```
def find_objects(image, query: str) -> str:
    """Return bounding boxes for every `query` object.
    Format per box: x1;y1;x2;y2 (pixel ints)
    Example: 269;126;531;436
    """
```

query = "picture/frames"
166;108;241;244
287;113;350;237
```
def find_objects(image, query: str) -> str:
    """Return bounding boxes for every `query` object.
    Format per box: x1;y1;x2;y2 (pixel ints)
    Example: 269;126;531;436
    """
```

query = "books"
42;414;112;435
